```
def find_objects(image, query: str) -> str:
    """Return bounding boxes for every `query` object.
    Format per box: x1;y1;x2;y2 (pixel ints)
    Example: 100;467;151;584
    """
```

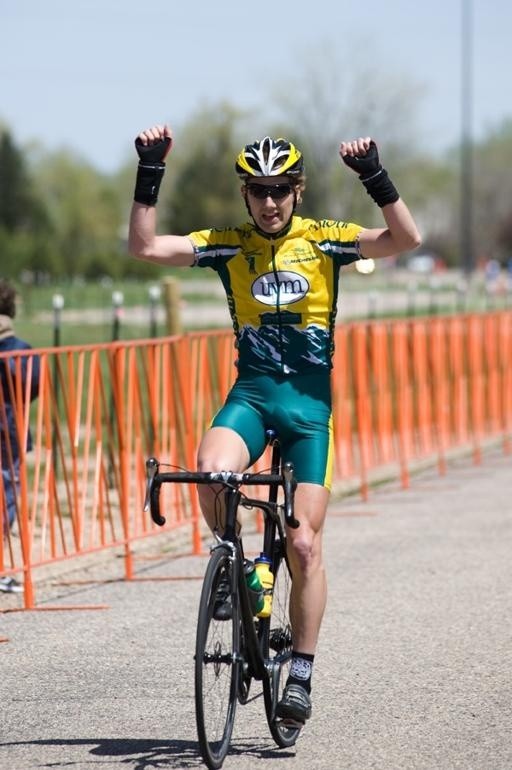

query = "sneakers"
0;574;26;594
273;681;314;732
212;563;236;622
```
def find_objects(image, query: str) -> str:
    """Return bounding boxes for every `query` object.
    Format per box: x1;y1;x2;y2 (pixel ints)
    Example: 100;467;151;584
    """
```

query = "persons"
125;122;425;722
2;280;44;593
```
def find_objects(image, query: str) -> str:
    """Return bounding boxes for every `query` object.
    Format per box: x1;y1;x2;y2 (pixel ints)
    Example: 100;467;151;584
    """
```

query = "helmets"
232;135;308;181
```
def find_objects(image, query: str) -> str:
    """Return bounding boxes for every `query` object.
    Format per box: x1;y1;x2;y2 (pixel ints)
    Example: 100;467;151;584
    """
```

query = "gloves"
134;133;173;166
341;139;384;182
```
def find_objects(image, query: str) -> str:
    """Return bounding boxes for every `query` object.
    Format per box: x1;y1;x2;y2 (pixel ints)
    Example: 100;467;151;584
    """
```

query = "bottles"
252;551;275;618
243;559;265;618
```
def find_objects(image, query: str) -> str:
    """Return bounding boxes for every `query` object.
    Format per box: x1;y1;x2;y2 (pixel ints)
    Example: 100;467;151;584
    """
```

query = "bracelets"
133;159;168;207
358;163;400;211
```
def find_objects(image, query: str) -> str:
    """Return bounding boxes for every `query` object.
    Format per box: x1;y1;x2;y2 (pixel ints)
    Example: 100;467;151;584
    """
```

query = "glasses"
243;178;296;199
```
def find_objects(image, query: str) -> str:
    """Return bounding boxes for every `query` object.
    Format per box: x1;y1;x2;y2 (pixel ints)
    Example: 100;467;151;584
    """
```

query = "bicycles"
140;428;300;770
